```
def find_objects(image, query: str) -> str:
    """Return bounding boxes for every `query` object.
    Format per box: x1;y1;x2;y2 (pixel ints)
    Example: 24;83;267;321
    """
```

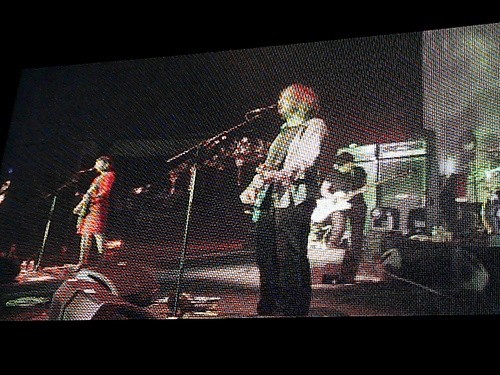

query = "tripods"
146;148;200;319
20;193;59;278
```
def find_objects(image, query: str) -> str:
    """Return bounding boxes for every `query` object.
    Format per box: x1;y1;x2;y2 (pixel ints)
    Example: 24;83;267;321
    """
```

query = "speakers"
49;263;164;321
339;138;431;242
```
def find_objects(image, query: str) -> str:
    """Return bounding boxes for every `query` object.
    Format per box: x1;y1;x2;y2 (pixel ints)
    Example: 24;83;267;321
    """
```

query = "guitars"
250;156;281;222
311;168;412;224
75;186;98;216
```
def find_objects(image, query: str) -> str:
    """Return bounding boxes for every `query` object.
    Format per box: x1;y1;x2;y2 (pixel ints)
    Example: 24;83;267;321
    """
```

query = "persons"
71;156;115;272
319;152;367;256
240;81;329;317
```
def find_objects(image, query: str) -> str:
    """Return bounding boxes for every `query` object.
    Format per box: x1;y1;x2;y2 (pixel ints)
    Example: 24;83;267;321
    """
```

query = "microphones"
86;166;95;172
249;102;278;114
